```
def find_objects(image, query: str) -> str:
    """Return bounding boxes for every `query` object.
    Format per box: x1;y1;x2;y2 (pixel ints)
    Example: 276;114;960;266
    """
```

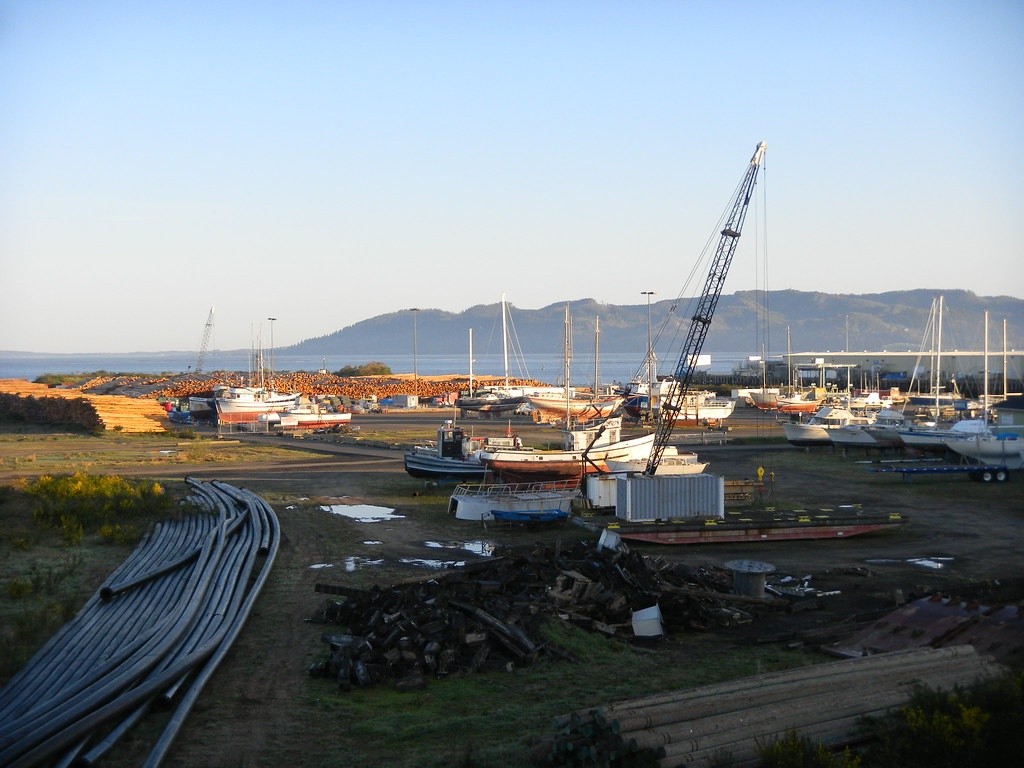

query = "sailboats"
748;294;1024;470
185;383;353;431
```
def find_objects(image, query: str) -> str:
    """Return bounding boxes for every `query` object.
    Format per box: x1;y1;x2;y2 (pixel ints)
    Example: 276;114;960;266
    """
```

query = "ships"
456;281;529;412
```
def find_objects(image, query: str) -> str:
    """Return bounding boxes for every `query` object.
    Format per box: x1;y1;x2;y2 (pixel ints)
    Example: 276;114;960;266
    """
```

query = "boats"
525;301;625;425
601;416;710;475
491;504;570;528
404;418;498;476
623;292;736;419
476;434;656;477
